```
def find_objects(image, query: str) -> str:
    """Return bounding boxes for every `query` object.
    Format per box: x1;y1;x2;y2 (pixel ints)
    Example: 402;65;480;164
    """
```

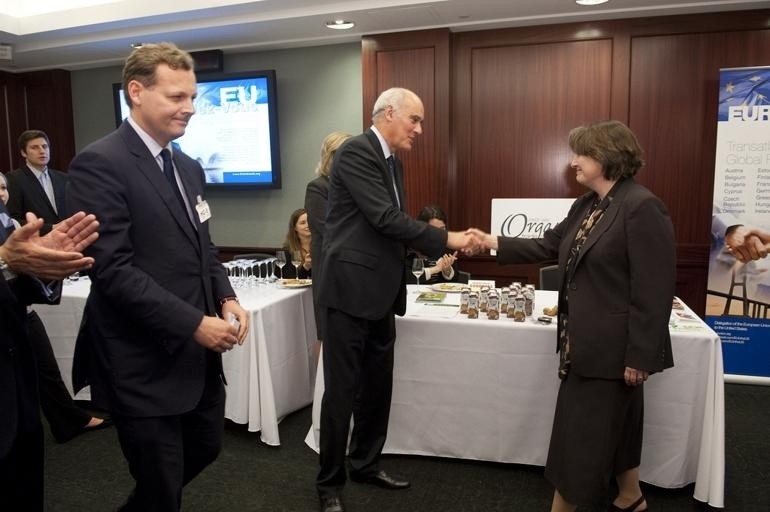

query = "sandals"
608;494;651;512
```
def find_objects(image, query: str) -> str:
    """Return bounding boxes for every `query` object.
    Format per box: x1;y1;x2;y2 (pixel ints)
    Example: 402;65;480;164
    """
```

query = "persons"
0;194;104;512
277;208;314;278
462;118;677;511
406;203;459;284
9;128;68;234
711;204;767;263
0;169;114;447
305;131;354;364
725;227;770;258
64;40;251;512
316;85;482;510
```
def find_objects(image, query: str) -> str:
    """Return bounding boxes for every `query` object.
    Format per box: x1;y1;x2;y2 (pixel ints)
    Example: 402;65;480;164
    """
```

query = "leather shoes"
81;414;114;433
348;470;411;491
318;496;345;512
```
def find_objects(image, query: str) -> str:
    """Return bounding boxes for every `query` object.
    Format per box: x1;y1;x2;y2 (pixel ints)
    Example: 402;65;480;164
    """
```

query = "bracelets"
220;296;240;305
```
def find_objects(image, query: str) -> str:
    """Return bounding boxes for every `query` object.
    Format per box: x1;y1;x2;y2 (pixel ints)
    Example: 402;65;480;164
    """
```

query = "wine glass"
276;251;289;282
219;257;277;298
291;248;305;283
411;258;426;293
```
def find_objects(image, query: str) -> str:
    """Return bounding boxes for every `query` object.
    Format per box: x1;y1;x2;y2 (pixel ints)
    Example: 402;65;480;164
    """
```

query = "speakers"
188;50;223;71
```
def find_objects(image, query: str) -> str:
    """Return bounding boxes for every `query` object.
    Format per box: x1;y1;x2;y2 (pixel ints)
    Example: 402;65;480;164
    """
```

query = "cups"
68;272;79;285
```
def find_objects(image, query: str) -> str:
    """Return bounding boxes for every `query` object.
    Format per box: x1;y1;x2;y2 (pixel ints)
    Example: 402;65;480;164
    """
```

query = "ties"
160;148;202;249
40;173;54;212
386;153;396;188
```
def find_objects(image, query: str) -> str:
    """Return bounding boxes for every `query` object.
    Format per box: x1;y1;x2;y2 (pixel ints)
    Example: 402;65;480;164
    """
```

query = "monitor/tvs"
111;70;281;189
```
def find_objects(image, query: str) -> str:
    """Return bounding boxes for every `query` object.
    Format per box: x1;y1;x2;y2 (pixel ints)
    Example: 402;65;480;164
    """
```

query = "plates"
278;278;313;288
432;282;468;292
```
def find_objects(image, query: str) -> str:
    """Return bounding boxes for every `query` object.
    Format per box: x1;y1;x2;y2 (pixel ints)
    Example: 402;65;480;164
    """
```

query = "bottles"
460;281;537;322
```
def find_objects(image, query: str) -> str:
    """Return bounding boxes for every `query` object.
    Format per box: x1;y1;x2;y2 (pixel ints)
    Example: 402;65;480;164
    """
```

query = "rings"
638;377;643;380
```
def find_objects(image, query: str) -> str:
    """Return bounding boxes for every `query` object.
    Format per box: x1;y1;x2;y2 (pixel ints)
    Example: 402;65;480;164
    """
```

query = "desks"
305;284;719;508
27;275;319;446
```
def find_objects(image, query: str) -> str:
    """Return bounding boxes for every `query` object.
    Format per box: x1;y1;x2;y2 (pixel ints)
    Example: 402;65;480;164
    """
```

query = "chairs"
458;271;471;284
233;253;275;278
539;265;559;291
